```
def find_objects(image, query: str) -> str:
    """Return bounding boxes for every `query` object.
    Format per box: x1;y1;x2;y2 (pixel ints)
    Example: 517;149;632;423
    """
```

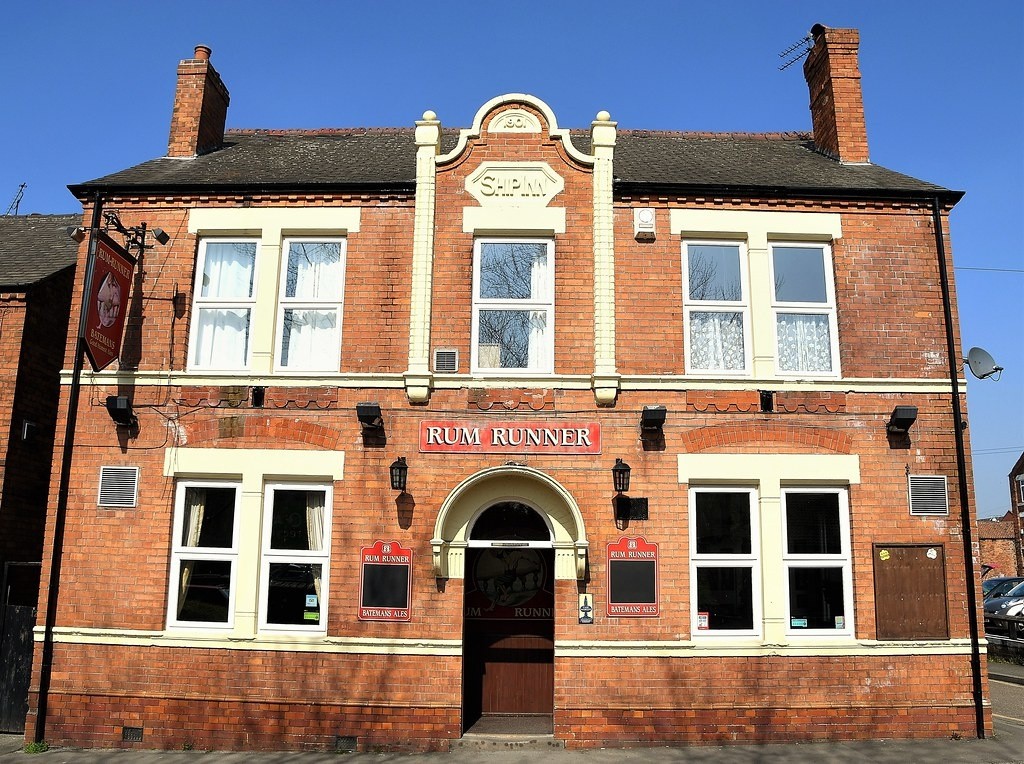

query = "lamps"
389;456;409;495
106;396;135;426
67;227;118;243
641;404;669;432
356;402;382;430
887;406;917;435
124;227;170;246
611;457;632;493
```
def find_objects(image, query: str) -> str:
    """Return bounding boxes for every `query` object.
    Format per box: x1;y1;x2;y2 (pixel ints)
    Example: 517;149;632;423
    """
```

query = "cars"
983;576;1024;635
